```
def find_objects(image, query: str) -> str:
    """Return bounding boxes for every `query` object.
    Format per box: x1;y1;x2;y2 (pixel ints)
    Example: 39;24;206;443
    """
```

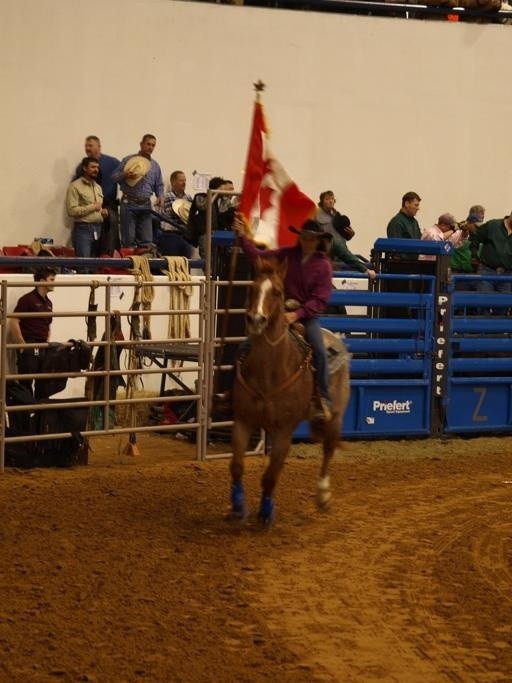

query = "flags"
237;100;318;250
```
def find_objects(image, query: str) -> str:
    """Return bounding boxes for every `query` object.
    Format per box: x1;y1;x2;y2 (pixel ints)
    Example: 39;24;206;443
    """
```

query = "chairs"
0;244;145;275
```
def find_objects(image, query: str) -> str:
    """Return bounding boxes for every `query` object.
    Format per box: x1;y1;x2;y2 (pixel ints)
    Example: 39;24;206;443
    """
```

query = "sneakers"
312;396;334;423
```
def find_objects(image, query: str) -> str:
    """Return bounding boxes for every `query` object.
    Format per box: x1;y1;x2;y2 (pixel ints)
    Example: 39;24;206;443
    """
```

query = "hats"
287;218;334;242
437;211;460;228
170;196;193;226
332;210;353;241
122;155;153;187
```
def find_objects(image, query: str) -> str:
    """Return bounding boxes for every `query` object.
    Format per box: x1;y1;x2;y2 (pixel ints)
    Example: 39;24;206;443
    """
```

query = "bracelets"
449;225;456;233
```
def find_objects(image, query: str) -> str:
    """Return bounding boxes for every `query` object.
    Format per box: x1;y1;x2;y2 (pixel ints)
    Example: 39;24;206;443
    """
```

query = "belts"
480;260;512;274
123;192;151;205
74;221;102;227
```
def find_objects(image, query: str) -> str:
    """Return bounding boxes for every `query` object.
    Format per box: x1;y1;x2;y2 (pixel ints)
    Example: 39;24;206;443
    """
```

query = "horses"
230;253;350;528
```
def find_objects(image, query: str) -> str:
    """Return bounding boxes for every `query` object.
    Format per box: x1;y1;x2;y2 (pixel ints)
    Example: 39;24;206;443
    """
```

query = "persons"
223;180;234;199
189;178;227;247
386;191;422;260
66;157;109;274
329;240;376;280
154;170;193;259
215;213;339;422
469;210;512;315
75;135;122;258
316;190;355;246
10;267;56;400
418;212;468;260
112;134;164;247
451;204;485;272
